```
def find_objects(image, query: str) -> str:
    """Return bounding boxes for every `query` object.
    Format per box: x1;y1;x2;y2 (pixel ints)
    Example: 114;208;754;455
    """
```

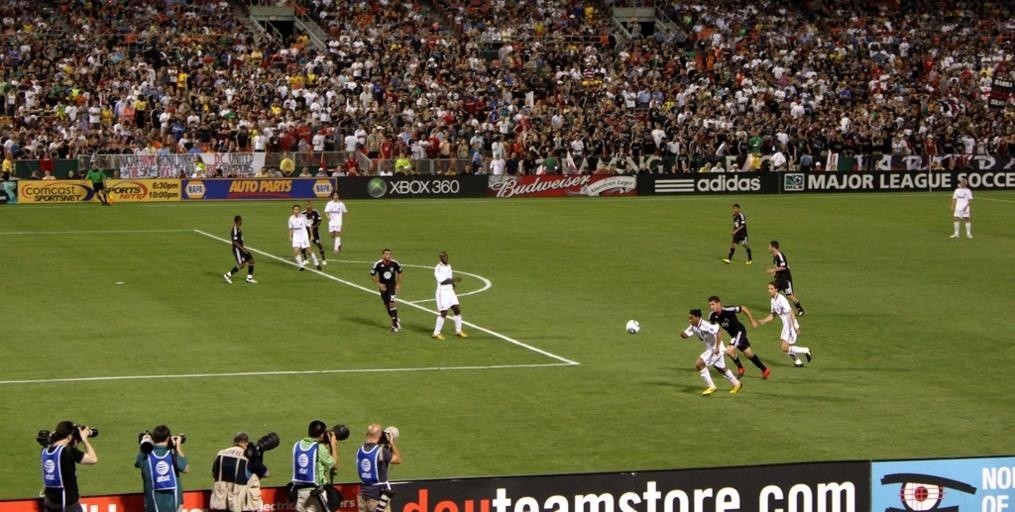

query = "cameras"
77;423;98;440
169;432;186;449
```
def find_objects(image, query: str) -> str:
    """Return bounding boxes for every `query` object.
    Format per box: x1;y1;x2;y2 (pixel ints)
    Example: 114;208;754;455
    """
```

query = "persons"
758;281;812;369
287;420;340;512
38;420;98;512
323;189;348;254
706;295;771;381
0;0;1014;182
766;240;805;317
431;250;468;340
206;431;271;512
132;425;191;511
355;423;402;512
722;203;753;266
369;248;404;334
679;308;744;395
287;205;322;271
946;177;974;240
220;215;258;285
300;200;328;266
84;162;109;206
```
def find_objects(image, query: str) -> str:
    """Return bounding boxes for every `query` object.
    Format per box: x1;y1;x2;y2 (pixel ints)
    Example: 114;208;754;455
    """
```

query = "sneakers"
806;347;812;362
391;326;398;332
798;310;804;317
299;260;327;271
455;331;467;338
746;260;753;265
950;233;961;239
246;277;258;283
792;364;803;368
966;234;973;239
702;367;771;396
432;334;444;340
722;258;732;264
223;274;232;285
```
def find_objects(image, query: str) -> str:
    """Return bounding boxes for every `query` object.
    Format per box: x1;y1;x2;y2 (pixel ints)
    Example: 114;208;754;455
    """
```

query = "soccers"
626;320;640;334
